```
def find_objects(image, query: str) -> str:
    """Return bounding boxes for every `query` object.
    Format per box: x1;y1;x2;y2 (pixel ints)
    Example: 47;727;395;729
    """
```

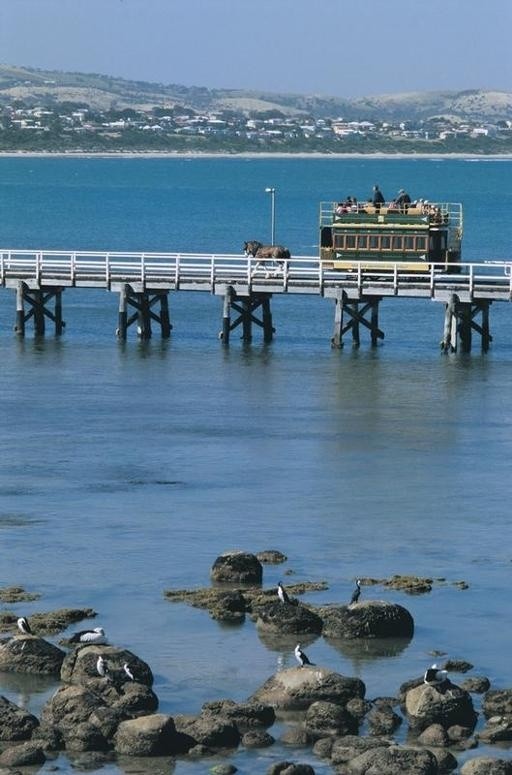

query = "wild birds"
295;641;315;668
123;660;142;683
69;627;105;646
277;580;291;605
17;616;32;635
350;578;362;605
424;663;449;687
97;655;110;678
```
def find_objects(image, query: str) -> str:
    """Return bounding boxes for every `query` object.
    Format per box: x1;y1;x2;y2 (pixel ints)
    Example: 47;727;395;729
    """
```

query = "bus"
318;200;463;281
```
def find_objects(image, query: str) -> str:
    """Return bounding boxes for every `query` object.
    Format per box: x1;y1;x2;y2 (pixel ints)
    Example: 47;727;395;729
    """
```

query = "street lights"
266;187;276;248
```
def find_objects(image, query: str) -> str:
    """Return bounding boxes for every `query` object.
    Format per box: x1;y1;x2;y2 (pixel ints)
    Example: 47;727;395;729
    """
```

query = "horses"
243;240;290;270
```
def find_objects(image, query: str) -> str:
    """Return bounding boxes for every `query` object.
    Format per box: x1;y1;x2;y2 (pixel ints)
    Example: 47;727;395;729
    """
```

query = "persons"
393;189;412;208
335;194;443;222
372;184;385;208
